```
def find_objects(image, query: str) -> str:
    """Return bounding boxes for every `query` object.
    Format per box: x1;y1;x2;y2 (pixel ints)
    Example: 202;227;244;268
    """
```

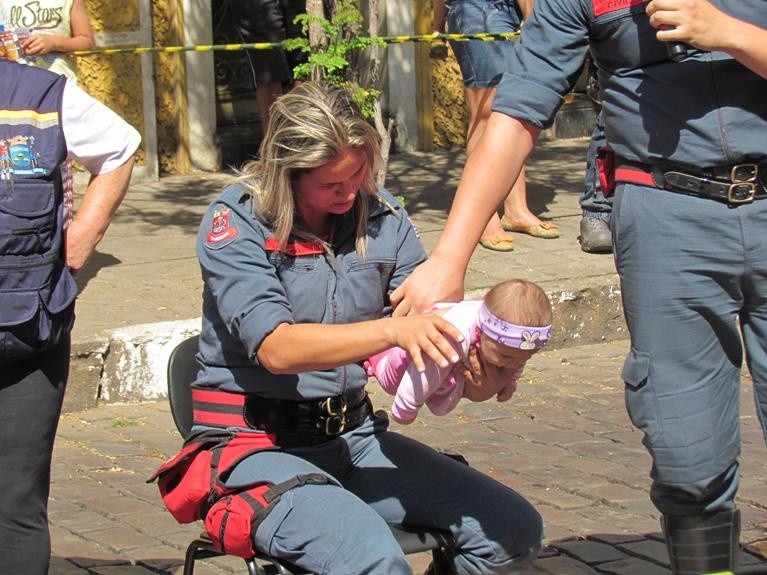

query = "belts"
251;381;369;444
654;161;767;205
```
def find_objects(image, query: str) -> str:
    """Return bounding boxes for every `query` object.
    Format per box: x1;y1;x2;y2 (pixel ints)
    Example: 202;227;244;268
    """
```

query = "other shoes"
500;214;560;239
480;233;513;251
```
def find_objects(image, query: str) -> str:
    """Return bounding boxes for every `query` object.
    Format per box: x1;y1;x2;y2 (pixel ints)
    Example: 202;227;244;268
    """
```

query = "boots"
661;508;740;575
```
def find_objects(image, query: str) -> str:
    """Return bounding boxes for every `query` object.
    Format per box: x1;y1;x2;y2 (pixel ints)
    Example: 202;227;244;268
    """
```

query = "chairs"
168;333;470;575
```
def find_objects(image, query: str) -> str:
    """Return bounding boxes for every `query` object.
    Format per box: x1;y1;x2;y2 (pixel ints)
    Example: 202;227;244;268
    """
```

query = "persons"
577;54;614;254
0;59;142;575
227;0;305;139
448;0;559;253
364;278;552;425
0;0;98;234
389;0;767;575
178;81;545;575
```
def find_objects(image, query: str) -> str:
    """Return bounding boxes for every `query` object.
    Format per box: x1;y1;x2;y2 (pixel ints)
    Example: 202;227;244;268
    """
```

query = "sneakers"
578;216;612;252
